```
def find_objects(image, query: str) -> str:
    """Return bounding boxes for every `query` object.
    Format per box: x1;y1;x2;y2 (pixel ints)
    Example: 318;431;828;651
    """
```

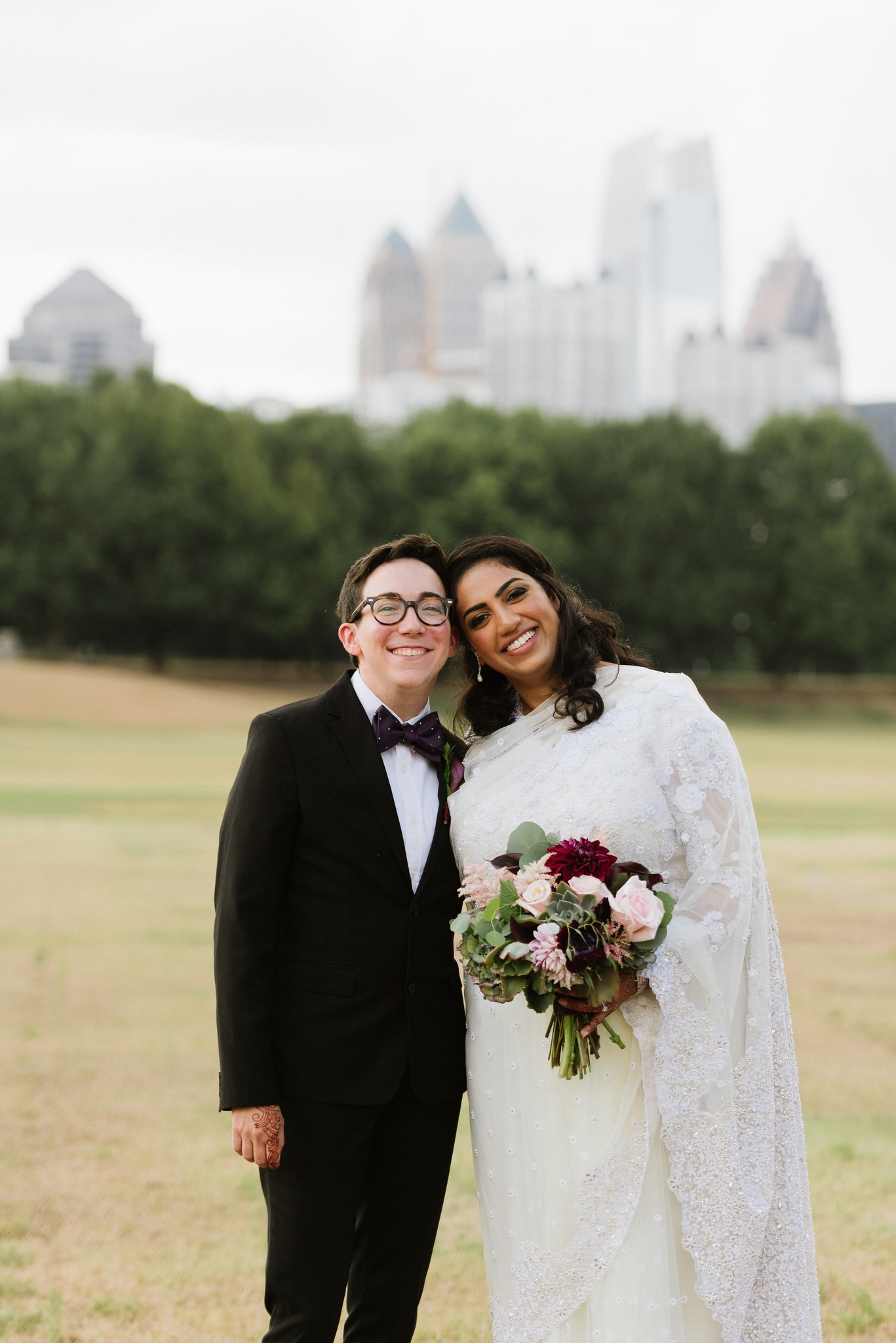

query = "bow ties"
370;706;444;765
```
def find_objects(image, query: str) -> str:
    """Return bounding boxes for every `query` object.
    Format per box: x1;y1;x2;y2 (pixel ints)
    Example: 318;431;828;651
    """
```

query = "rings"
601;1001;608;1013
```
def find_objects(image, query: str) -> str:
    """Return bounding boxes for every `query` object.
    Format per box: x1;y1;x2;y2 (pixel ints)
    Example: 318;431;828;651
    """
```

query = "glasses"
348;593;454;627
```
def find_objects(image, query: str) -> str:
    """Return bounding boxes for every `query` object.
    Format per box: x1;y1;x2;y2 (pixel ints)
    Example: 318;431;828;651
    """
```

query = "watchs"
632;969;648;996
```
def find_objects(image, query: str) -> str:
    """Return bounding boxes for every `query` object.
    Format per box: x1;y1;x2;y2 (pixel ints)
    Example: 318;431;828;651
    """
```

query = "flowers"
446;820;677;1083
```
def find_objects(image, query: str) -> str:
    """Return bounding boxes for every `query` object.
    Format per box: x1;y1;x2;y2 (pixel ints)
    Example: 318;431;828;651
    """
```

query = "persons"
439;538;825;1343
213;532;469;1343
